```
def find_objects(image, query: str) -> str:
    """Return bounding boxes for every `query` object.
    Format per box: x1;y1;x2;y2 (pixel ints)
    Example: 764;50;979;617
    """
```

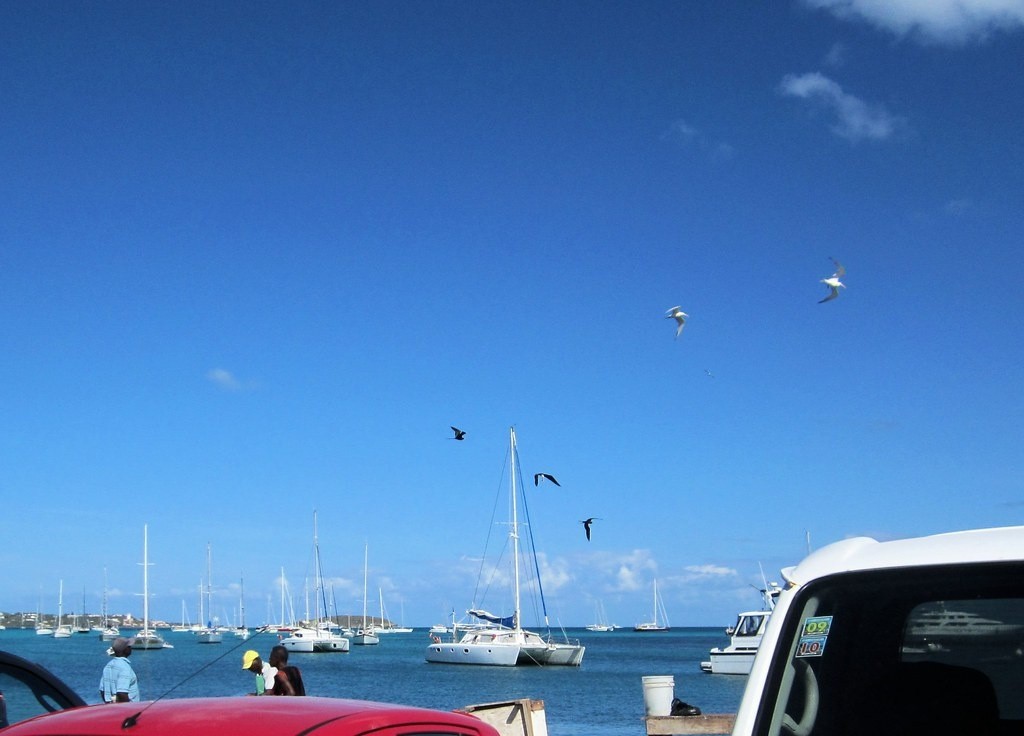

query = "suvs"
729;526;1024;736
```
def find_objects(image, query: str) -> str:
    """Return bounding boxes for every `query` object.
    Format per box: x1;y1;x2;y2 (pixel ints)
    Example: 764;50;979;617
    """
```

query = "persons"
242;649;273;697
100;637;139;703
265;646;307;696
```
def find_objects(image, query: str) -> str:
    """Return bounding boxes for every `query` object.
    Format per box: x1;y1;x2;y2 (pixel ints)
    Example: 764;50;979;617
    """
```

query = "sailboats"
255;509;414;653
35;567;119;641
170;542;250;643
634;577;672;632
424;425;586;667
585;597;622;632
131;523;165;650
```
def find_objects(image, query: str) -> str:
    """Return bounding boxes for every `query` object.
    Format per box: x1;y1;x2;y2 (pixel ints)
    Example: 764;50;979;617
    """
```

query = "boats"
700;560;783;675
905;599;1023;658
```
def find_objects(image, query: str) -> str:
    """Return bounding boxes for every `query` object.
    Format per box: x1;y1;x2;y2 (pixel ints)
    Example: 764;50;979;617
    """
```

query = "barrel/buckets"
641;676;676;716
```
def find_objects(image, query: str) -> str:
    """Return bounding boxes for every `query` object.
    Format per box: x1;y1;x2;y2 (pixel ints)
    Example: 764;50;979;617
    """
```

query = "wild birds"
665;306;690;338
445;424;467;441
578;517;604;542
534;472;562;488
816;256;851;305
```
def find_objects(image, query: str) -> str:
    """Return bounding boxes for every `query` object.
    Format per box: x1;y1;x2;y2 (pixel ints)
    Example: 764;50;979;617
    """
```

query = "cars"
0;695;502;736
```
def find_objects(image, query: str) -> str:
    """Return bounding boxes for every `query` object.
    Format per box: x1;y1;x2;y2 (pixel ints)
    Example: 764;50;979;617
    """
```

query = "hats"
112;637;135;653
242;650;259;669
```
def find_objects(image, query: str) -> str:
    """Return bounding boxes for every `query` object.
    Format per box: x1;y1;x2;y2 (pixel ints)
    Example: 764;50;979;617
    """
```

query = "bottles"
256;673;267;696
